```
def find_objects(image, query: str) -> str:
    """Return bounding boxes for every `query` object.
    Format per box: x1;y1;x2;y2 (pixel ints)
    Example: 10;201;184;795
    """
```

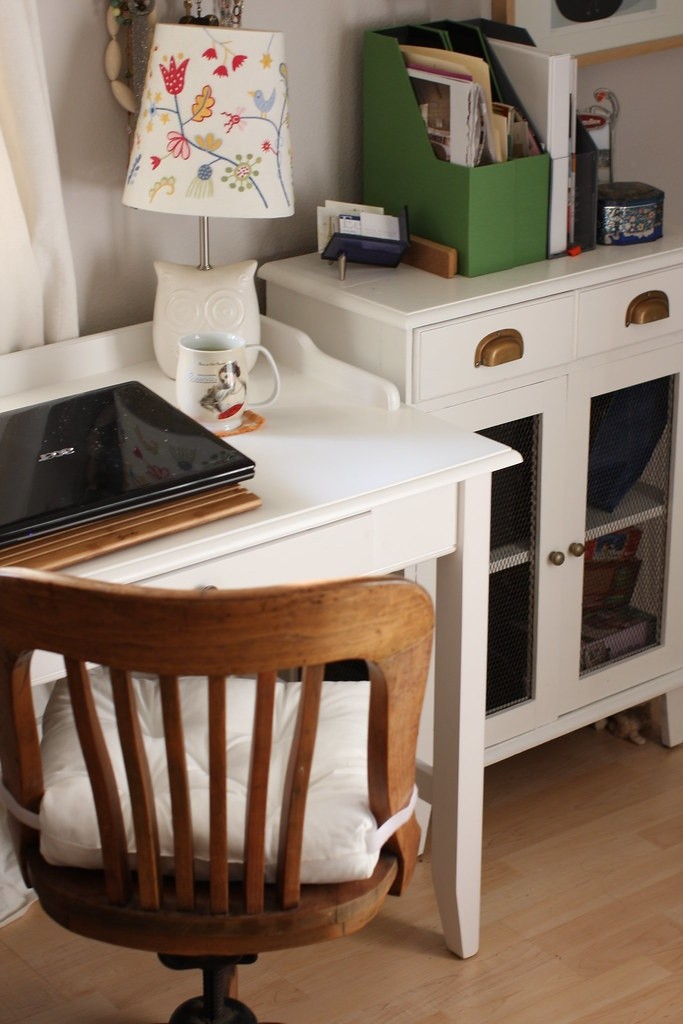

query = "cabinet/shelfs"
257;235;683;769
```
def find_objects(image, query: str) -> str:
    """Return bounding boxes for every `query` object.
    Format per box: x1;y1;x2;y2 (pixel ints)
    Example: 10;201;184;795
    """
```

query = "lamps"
119;21;296;379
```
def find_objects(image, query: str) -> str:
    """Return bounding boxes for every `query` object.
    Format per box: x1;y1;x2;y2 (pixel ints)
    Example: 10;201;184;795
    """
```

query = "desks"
0;307;525;959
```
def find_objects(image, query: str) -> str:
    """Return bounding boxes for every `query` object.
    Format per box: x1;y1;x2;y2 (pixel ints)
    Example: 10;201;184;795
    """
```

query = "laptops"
2;380;257;546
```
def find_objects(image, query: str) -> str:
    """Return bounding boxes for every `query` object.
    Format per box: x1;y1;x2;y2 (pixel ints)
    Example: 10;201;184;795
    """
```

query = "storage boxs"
359;15;600;282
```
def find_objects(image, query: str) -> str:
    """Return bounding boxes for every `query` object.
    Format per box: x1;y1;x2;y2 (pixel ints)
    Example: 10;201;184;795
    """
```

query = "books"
480;34;578;257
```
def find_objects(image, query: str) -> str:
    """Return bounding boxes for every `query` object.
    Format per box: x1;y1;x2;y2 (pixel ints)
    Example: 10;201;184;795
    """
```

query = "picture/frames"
490;0;683;66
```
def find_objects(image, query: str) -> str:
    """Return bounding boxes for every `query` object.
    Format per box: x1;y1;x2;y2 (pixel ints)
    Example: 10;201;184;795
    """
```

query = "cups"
176;330;279;435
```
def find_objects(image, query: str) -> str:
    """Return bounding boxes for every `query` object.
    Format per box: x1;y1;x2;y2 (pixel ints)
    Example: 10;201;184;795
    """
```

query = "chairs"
0;566;435;1024
